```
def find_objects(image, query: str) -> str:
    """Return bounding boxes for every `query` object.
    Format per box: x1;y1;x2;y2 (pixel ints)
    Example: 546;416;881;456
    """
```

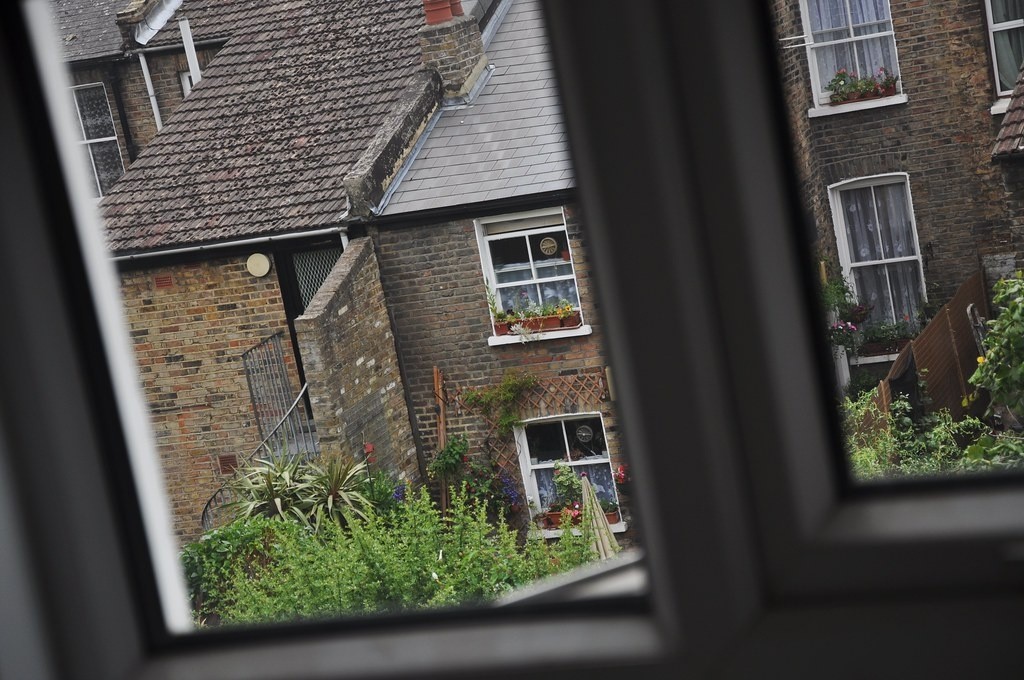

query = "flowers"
898;314;911;336
879;66;899;86
612;464;628;481
864;73;881;92
548;503;563;512
830;320;858;347
514;302;556;316
848;72;860;89
558;302;575;318
567;497;583;518
827;69;846;90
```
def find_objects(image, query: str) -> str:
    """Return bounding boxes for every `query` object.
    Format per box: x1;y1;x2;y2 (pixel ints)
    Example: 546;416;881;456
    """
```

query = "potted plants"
485;277;508;336
860;323;895;354
599;500;619;525
530;500;548;529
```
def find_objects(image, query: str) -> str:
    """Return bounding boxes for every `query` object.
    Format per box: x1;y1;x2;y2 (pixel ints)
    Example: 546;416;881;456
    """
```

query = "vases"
550;513;566;528
512;317;560;331
883;84;896;96
848;91;861;101
829;92;845;102
865;90;877;99
898;339;913;351
615;480;630;496
562;311;581;327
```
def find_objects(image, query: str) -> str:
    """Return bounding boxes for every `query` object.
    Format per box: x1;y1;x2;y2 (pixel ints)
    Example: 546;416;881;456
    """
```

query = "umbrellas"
580;472;622;561
